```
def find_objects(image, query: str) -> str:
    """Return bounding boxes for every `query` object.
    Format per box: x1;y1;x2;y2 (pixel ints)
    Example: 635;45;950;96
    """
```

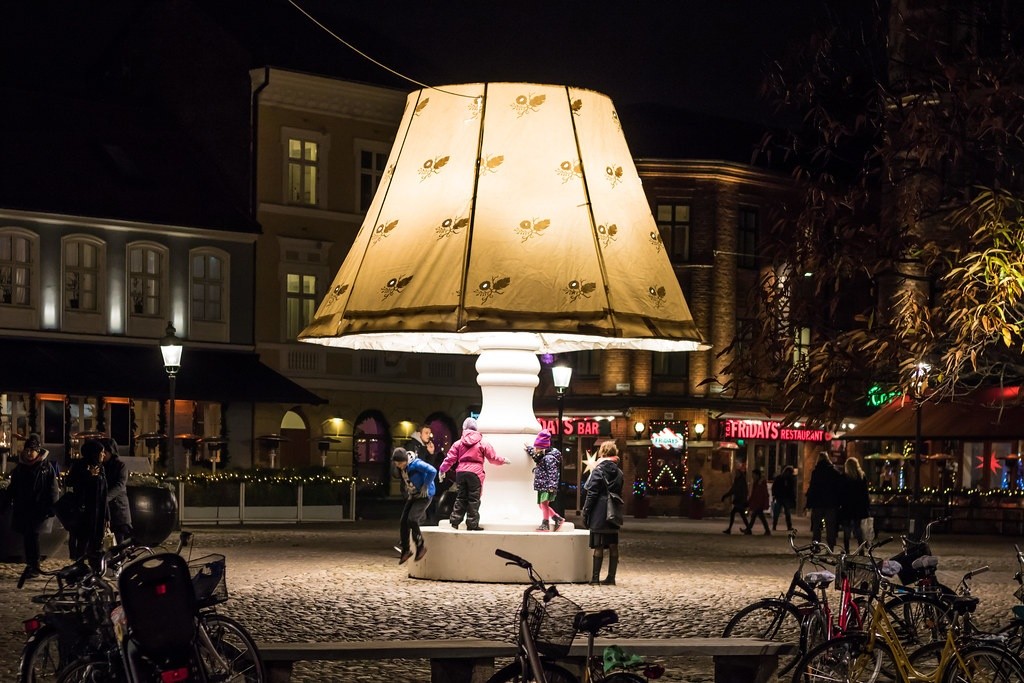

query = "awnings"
0;335;323;405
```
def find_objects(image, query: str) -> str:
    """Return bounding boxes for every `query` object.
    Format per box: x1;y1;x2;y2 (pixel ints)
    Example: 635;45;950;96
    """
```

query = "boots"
536;519;550;532
602;556;618;584
414;540;427;560
589;555;603;585
552;512;566;532
399;550;413;565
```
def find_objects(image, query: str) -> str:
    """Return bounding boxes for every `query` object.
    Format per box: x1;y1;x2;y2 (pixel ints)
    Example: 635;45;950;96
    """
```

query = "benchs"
236;639;793;683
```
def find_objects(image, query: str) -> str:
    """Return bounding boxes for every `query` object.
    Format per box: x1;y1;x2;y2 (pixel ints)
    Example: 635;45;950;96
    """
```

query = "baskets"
834;554;883;594
513;591;585;659
1014;584;1024;603
890;544;937;586
185;553;228;608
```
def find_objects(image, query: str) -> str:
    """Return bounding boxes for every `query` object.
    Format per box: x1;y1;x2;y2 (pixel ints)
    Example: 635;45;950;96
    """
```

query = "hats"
534;429;552;449
462;417;477;431
81;441;105;462
390;447;408;462
24;433;41;451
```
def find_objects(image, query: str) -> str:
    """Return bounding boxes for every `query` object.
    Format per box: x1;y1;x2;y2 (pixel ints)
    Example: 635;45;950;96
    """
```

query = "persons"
438;417;510;530
582;440;624;585
936;460;952;490
0;433;59;584
807;451;872;556
880;465;896;497
65;437;133;573
524;428;565;531
393;423;459;554
390;447;437;565
721;466;797;536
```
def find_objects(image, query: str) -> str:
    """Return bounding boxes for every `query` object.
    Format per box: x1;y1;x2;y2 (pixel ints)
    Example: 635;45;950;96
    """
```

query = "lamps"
297;81;718;582
692;423;706;440
633;421;645;439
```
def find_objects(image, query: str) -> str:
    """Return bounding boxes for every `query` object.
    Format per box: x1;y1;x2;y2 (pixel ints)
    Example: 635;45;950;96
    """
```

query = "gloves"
503;458;511;464
418;484;429;498
439;471;445;483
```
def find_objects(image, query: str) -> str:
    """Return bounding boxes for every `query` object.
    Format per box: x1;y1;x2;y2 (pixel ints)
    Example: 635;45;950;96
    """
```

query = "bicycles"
485;549;667;683
715;535;1024;683
9;524;268;683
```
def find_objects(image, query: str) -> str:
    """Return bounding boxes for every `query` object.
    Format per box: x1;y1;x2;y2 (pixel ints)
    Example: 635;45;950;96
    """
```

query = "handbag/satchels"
861;517;875;542
55;491;84;527
770;498;776;517
606;492;624;526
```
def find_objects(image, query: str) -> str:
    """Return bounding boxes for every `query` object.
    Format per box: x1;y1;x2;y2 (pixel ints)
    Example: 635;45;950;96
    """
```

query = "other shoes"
467;526;484;531
723;523;795;536
452;524;459;529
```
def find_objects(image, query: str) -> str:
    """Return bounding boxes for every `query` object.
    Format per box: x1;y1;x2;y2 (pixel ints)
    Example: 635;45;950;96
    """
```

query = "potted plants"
132;276;144;314
632;474;650;519
0;274;10;304
66;271;80;308
686;472;705;521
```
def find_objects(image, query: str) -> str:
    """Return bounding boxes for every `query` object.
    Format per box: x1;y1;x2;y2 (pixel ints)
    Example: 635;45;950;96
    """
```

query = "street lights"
551;361;582;520
158;319;185;483
907;360;933;522
